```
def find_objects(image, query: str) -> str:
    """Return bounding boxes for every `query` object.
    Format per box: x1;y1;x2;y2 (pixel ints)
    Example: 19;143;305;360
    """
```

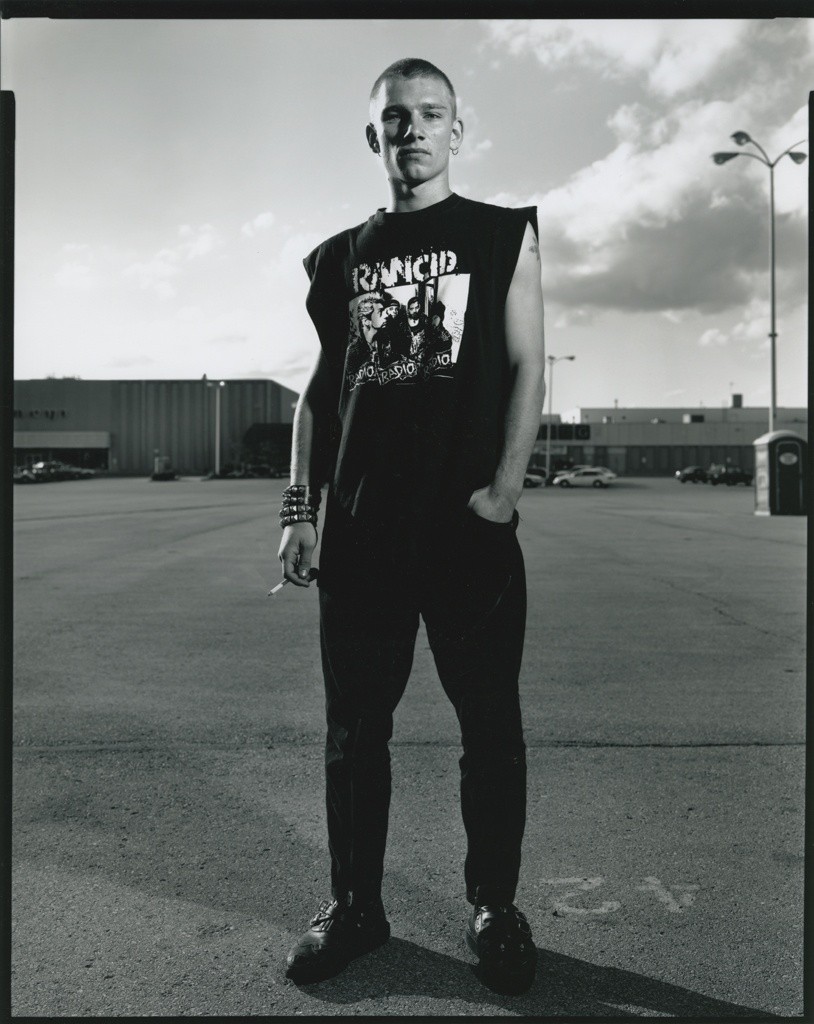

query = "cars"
13;460;84;484
553;467;617;488
523;473;543;489
553;464;594;477
675;465;709;484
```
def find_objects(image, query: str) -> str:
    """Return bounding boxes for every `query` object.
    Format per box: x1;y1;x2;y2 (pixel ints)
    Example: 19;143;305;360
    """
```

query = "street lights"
206;381;225;476
707;129;808;435
545;354;574;480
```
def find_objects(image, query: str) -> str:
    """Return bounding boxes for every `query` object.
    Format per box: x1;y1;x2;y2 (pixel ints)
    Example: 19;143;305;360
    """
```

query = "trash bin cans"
155;456;171;474
752;429;806;516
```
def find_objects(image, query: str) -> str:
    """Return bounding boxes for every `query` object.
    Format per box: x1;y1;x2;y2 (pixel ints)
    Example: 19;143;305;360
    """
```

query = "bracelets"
279;484;322;529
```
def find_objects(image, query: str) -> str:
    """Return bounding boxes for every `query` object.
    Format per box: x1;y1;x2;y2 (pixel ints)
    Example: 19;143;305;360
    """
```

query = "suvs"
710;463;753;486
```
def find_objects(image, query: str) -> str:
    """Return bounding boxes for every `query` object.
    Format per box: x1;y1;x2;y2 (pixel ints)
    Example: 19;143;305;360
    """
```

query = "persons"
274;55;546;994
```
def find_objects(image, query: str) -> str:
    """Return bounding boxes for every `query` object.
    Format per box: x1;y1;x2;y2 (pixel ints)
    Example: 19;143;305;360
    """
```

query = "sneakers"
466;900;533;998
283;891;398;986
